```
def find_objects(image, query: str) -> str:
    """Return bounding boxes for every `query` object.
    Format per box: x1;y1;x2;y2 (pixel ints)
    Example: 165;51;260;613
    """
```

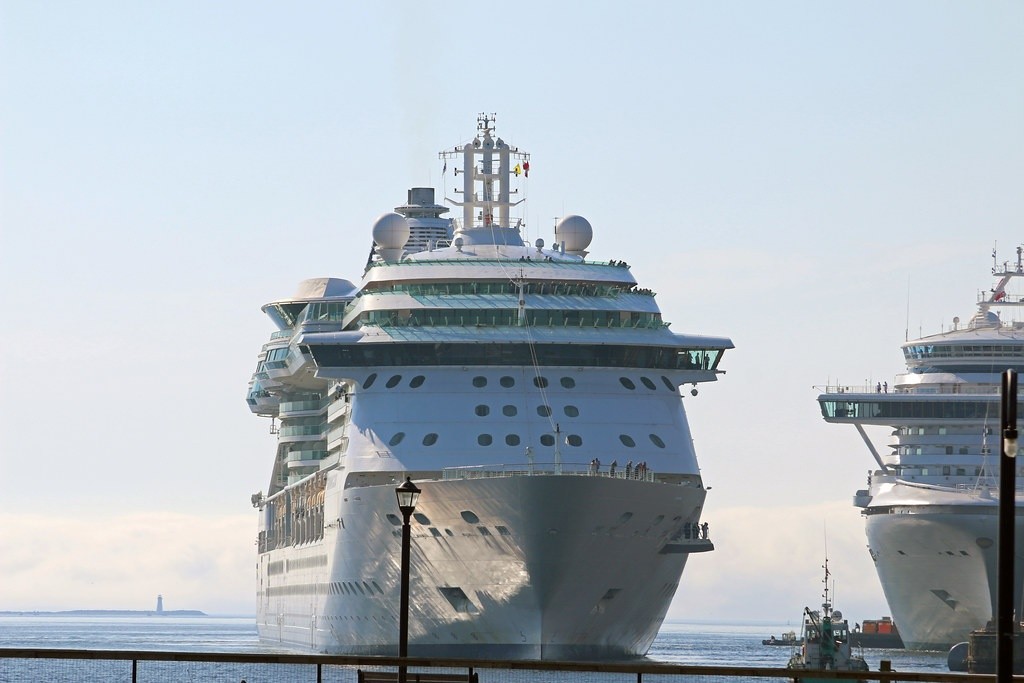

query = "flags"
994;291;1007;301
513;163;521;176
523;161;529;177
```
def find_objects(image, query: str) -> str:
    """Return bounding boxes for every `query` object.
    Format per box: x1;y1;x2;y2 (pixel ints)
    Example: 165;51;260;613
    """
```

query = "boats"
786;521;876;683
762;631;804;648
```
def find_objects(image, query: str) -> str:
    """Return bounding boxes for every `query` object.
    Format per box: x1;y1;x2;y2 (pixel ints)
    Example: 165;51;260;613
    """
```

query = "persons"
609;459;617;478
519;255;532;262
627;287;657;297
701;522;709;538
695;353;710;370
484;209;493;227
589;457;600;476
543;256;556;263
609;259;627;267
634;461;647;480
878;382;881;393
883;381;888;394
624;461;633;480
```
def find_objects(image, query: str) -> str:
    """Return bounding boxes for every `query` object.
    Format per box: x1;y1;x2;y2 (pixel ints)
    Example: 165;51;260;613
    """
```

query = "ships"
812;237;1024;653
250;111;737;659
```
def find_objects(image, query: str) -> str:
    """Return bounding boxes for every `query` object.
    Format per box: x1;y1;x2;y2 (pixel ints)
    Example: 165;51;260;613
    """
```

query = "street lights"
997;367;1019;683
396;474;421;683
